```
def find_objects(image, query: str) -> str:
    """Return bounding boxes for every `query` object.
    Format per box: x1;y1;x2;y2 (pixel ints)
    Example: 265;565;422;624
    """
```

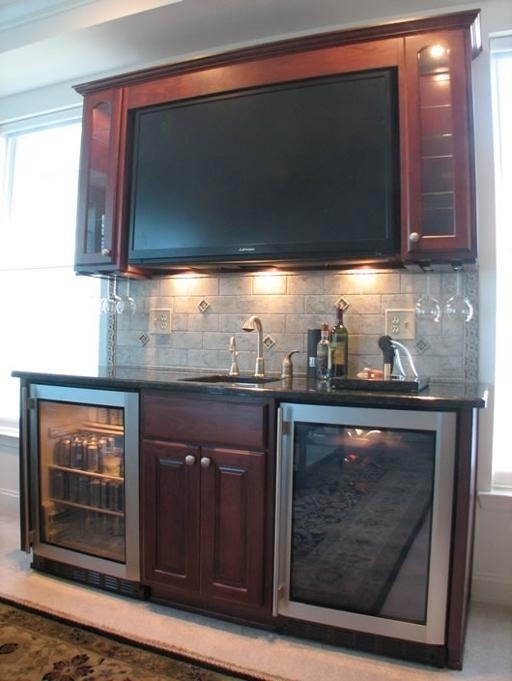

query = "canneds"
54;433;124;536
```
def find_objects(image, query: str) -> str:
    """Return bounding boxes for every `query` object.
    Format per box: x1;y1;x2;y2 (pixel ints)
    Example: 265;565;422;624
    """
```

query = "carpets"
290;431;433;616
0;591;295;681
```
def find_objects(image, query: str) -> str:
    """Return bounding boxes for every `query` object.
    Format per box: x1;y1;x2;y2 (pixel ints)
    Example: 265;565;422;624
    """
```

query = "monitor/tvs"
127;69;392;267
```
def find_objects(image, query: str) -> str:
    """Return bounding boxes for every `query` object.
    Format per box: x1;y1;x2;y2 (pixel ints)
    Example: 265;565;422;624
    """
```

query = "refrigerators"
17;382;144;584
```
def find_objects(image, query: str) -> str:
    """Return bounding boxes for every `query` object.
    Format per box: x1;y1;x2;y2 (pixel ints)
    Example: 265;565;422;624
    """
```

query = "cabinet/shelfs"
139;390;274;631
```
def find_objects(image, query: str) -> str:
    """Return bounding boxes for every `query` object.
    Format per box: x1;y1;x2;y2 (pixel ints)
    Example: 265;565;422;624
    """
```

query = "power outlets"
385;309;416;340
148;308;172;335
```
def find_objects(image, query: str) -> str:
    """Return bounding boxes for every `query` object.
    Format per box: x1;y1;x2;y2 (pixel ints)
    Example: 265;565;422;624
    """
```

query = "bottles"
306;328;320;378
48;433;123;543
316;324;330;378
330;303;349;378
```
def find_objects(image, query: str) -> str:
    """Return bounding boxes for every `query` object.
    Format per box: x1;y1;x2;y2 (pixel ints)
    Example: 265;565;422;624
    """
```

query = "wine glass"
412;266;473;327
101;276;136;318
